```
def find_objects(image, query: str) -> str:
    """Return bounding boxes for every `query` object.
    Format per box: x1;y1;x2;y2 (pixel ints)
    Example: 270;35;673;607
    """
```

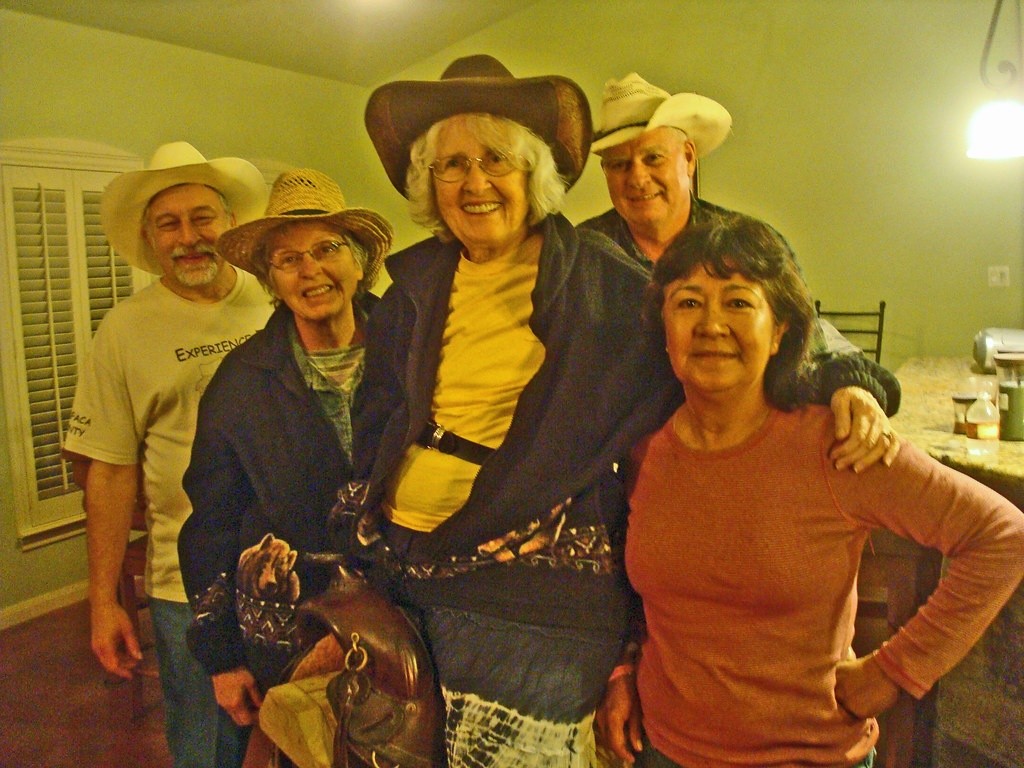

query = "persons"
177;170;394;768
574;74;798;273
596;215;1024;768
348;56;900;768
65;141;270;768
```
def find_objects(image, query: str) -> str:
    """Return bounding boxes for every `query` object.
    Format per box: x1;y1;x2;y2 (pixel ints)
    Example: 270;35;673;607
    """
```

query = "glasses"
428;150;518;183
264;241;348;275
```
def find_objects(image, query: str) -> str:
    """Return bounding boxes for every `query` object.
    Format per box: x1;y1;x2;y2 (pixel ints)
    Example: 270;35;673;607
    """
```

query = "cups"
952;392;978;434
998;381;1024;441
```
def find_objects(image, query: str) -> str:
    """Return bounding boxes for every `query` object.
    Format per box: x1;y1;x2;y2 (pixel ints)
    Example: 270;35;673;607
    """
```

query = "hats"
589;72;733;159
363;55;593;194
100;140;269;277
215;168;394;292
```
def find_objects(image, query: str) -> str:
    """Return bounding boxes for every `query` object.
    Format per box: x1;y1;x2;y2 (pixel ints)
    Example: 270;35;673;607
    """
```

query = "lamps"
964;0;1024;159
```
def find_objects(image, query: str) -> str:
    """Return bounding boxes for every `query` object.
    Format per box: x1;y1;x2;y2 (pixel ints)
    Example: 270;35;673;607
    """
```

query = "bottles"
965;392;1000;456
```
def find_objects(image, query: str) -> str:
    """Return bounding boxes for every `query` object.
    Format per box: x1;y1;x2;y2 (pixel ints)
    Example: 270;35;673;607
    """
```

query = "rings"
882;432;892;443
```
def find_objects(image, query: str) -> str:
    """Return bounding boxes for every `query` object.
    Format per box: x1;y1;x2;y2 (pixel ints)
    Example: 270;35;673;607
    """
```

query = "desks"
874;356;1024;768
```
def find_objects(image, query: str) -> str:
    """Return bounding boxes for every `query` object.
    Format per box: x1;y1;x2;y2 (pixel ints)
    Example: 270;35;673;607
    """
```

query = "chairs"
59;448;162;721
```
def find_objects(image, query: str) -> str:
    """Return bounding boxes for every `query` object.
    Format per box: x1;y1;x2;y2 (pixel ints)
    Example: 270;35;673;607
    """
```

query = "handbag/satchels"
286;561;444;768
